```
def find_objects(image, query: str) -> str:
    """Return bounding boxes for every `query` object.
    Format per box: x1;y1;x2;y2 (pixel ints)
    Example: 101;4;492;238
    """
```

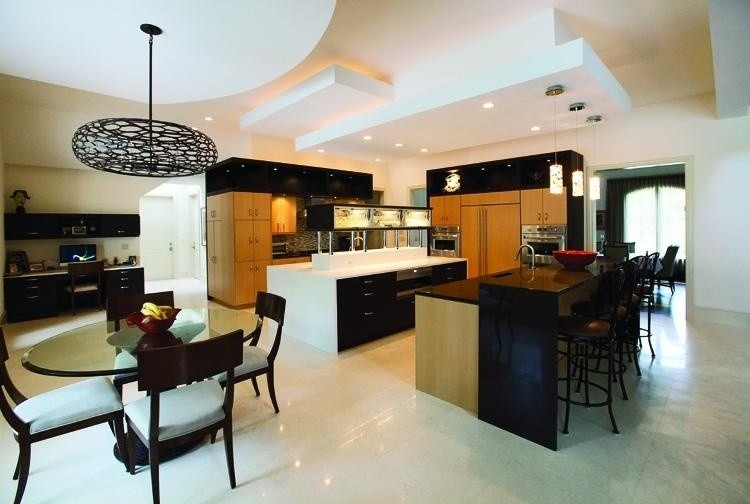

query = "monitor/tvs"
60;245;97;269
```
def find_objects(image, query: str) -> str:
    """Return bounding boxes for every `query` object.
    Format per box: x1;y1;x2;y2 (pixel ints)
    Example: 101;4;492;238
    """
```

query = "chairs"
611;252;660;357
106;292;176;357
557;268;626;434
0;327;128;504
655;245;680;295
567;261;638;401
121;328;243;504
209;290;286;444
64;262;105;312
592;256;651;376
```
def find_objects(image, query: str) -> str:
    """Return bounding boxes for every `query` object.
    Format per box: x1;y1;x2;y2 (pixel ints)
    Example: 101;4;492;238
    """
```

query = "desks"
4;264;143;321
20;310;263;376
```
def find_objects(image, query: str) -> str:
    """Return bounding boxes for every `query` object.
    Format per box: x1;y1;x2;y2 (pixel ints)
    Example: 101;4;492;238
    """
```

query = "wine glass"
600;233;604;246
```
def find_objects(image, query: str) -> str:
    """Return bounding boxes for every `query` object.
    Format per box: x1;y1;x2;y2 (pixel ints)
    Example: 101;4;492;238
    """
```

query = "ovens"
522;225;566;265
429;225;460;257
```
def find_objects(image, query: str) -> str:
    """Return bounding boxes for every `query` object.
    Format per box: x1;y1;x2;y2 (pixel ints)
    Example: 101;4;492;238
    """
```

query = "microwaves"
272;242;289;254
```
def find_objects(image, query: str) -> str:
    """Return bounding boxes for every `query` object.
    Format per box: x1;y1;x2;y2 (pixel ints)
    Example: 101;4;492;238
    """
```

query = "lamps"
71;23;217;178
586;115;602;198
546;86;565;194
568;103;584;198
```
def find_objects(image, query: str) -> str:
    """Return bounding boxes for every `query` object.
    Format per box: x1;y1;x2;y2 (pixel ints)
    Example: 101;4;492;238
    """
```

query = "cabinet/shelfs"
429;195;460;227
338;272;395;350
521;188;566;224
273;257;311;264
4;213;140;240
396;292;430;332
207;192;272;310
431;260;466;284
460;190;520;279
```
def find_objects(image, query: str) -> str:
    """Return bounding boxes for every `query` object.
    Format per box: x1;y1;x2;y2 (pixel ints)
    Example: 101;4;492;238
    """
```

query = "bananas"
140;302;172;319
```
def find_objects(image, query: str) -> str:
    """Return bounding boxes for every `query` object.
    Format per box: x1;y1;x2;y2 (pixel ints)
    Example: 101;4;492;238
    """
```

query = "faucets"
514;245;537;268
519;268;535;284
348;237;364;252
